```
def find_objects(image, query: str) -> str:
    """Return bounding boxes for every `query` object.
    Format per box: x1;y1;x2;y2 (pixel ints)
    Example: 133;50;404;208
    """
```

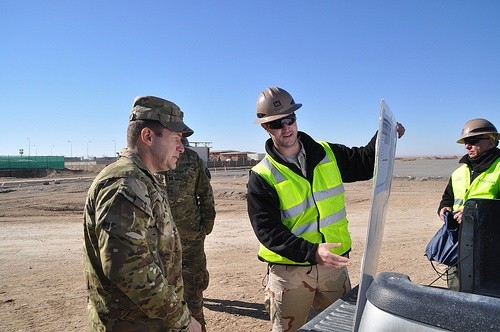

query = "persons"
438;118;500;290
83;95;201;332
247;87;406;332
157;147;216;332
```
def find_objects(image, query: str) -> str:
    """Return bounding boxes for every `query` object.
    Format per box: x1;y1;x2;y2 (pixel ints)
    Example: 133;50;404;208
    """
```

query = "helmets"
255;86;304;123
456;119;497;143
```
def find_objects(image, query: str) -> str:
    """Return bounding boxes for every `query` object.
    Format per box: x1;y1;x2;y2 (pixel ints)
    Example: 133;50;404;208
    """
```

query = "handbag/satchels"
425;210;463;280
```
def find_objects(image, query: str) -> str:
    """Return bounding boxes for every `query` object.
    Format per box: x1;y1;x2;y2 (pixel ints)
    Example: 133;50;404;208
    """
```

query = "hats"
129;95;194;137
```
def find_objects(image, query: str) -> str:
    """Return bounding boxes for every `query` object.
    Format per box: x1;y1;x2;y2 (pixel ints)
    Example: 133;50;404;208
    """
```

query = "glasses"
268;114;297;128
464;137;491;145
